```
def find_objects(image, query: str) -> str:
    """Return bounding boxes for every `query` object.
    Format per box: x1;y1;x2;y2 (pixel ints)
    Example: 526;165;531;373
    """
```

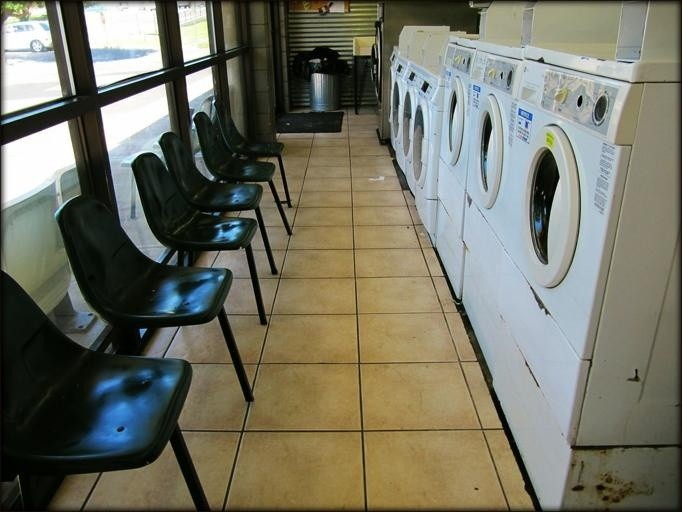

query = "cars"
4;21;54;52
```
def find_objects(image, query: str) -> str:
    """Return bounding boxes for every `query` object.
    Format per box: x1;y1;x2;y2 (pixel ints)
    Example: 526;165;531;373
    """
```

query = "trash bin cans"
309;59;340;112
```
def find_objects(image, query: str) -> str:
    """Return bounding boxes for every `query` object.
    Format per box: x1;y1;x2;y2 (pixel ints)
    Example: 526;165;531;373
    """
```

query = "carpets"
274;113;345;133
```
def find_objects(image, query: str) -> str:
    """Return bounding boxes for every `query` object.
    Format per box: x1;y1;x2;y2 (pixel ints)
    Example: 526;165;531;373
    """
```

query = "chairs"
193;112;293;237
55;193;254;404
159;131;280;274
130;153;271;325
214;98;293;208
0;270;213;512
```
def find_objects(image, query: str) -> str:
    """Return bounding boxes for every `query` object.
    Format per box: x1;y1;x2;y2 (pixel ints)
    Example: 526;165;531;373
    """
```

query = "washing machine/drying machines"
385;2;682;512
